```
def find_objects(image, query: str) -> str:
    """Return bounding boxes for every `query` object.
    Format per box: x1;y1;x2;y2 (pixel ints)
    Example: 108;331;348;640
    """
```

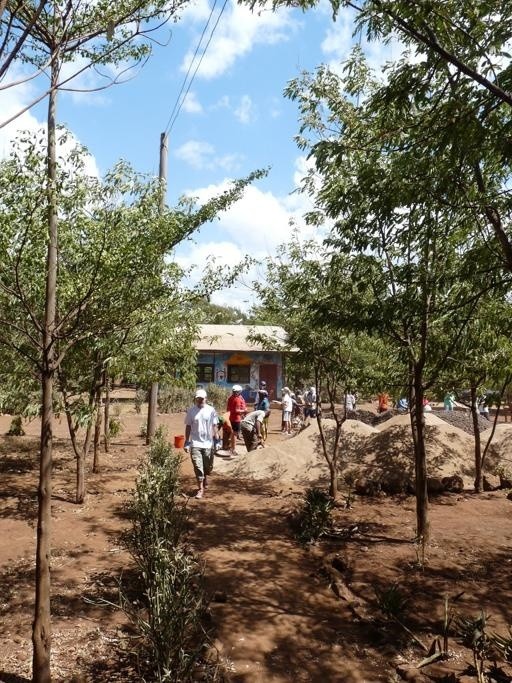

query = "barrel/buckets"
174;435;186;448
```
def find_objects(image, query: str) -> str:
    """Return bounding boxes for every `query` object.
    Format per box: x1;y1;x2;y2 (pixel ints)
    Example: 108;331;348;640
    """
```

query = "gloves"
184;441;191;453
213;440;221;451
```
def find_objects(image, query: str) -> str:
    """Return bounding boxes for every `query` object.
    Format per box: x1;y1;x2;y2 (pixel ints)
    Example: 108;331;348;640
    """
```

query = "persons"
216;381;317;464
343;389;359;412
370;388;490;422
183;389;221;498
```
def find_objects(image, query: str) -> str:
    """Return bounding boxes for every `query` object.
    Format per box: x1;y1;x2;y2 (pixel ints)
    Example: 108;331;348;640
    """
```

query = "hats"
232;384;242;392
281;386;289;391
195;389;206;399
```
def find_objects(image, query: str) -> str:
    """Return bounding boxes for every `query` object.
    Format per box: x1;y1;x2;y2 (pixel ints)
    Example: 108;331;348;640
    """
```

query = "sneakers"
195;489;204;499
